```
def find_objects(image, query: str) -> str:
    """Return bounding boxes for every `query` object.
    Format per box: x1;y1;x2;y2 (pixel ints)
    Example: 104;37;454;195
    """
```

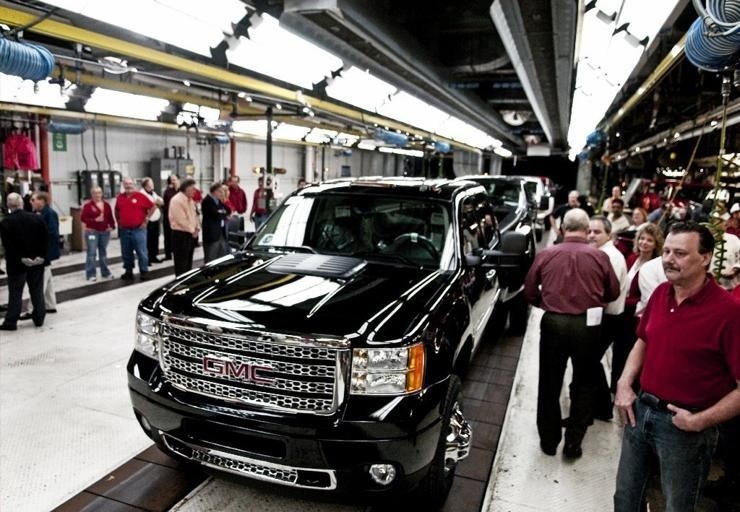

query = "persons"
1;182;60;330
80;186;115;282
114;174;274;281
298;179;306;188
524;182;739;512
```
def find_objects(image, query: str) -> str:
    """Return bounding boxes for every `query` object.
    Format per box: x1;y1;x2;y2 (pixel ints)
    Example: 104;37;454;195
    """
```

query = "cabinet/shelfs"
71;206;89;253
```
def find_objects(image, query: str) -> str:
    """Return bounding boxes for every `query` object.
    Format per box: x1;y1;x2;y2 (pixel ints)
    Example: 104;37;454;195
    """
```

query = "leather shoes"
0;321;17;331
17;310;34;321
562;443;584;461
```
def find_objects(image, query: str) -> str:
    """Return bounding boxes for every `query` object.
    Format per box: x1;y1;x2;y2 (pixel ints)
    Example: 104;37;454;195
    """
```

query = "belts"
638;390;683;415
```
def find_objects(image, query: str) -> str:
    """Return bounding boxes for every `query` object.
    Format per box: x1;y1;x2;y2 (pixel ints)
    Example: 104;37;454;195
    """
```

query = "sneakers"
101;274;115;280
88;276;97;282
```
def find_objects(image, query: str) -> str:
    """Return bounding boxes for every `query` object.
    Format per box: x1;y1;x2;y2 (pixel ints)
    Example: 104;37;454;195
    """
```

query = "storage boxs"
58;215;74;236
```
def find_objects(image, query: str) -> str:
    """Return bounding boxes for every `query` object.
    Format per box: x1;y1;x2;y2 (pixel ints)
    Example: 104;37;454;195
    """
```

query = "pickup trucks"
451;175;550;252
504;172;565;244
127;175;534;495
